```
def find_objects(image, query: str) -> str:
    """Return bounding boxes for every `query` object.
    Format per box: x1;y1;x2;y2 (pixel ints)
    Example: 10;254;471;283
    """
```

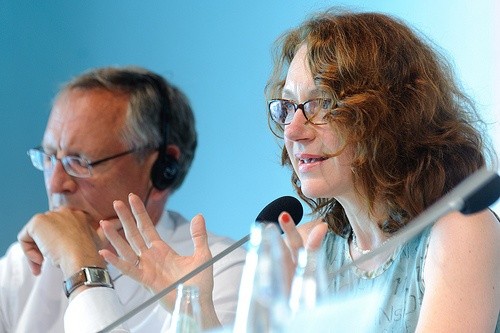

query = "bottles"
233;221;289;333
285;247;332;333
170;283;202;333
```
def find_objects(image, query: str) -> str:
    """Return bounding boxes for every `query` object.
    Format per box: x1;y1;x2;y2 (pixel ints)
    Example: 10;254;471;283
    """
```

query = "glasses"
267;99;332;125
26;146;150;178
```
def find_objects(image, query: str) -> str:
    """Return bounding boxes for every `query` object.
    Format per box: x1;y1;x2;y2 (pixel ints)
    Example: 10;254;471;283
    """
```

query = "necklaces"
352;232;395;255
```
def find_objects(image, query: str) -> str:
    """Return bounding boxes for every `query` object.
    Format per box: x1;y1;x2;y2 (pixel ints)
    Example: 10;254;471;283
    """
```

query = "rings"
135;257;140;265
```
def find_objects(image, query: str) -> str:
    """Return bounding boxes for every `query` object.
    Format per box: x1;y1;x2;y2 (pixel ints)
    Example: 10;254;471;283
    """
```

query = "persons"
0;67;247;333
98;4;500;333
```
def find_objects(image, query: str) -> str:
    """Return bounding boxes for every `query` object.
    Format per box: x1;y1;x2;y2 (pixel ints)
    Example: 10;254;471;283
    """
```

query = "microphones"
95;196;303;333
273;173;499;333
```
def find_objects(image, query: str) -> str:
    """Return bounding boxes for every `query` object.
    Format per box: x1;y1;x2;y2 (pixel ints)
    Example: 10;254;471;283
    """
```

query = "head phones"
148;71;181;192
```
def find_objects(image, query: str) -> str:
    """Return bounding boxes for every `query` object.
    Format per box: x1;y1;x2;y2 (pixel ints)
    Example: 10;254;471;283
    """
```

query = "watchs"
63;266;114;298
